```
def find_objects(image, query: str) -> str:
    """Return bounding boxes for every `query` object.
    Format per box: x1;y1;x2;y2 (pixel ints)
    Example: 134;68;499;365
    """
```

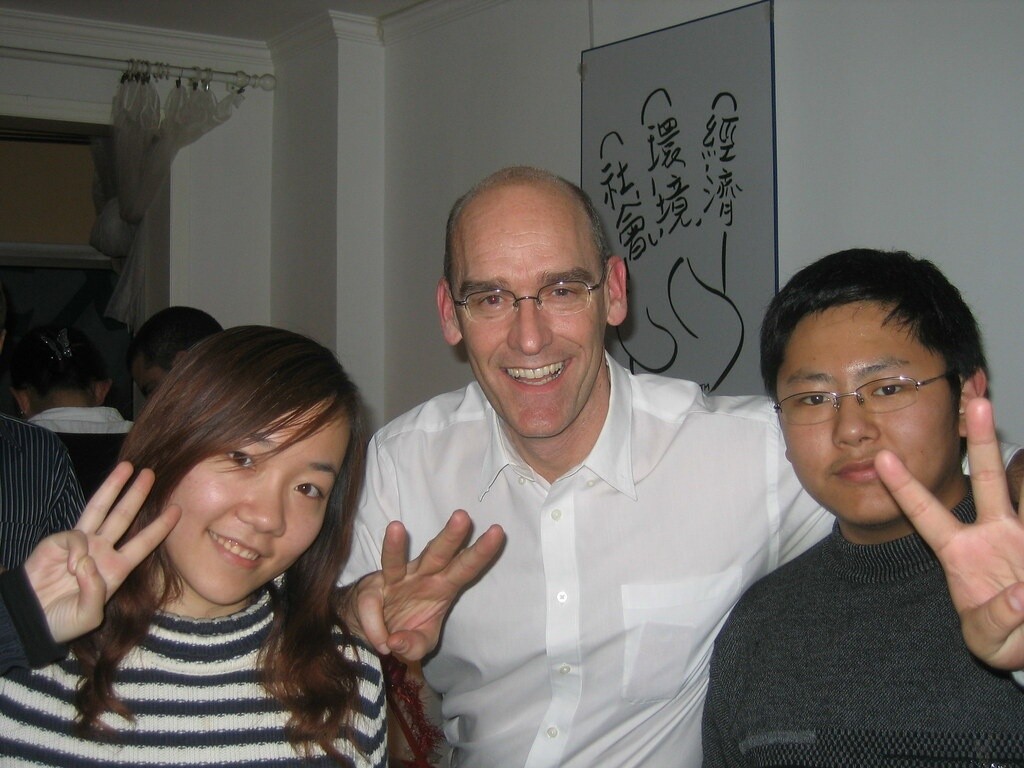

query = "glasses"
453;262;606;322
773;373;946;425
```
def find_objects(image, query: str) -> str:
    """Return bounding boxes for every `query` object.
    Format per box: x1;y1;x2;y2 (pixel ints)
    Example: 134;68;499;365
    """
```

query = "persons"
128;307;221;396
0;325;389;767
330;168;1024;767
699;249;1024;766
0;292;87;574
10;319;134;499
